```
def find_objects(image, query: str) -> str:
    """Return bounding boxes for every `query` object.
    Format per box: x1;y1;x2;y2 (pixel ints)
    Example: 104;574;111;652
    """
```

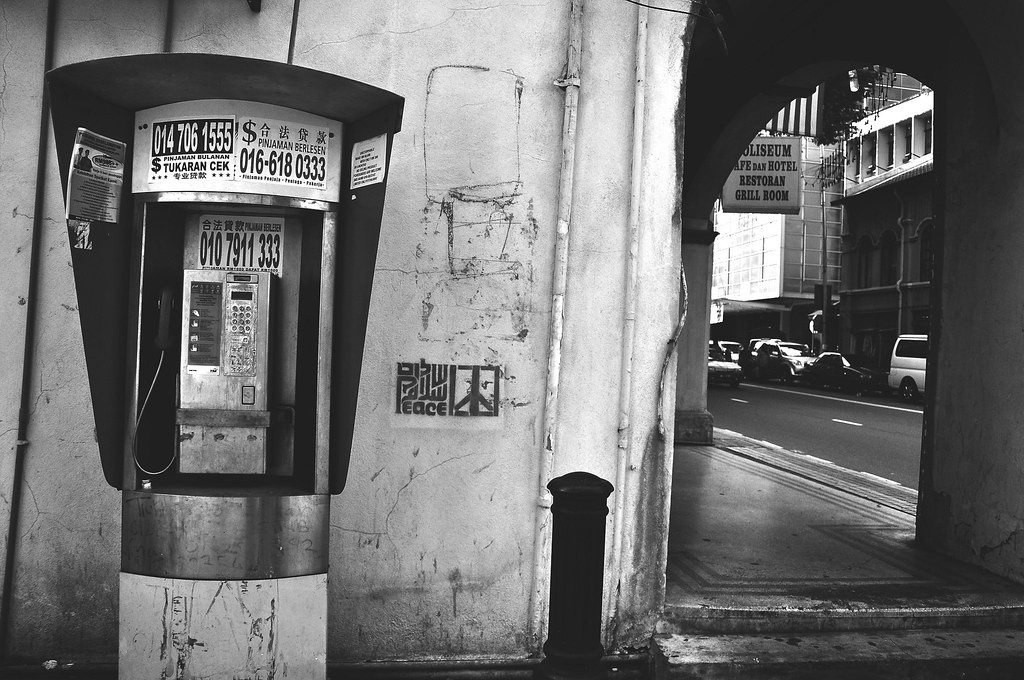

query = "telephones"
152;265;270;475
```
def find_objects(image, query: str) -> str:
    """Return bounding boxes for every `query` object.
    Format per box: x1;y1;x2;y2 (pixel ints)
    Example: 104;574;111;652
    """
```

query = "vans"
887;334;928;403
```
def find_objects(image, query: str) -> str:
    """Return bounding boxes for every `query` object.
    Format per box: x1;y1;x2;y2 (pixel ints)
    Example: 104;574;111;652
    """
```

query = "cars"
708;337;873;396
